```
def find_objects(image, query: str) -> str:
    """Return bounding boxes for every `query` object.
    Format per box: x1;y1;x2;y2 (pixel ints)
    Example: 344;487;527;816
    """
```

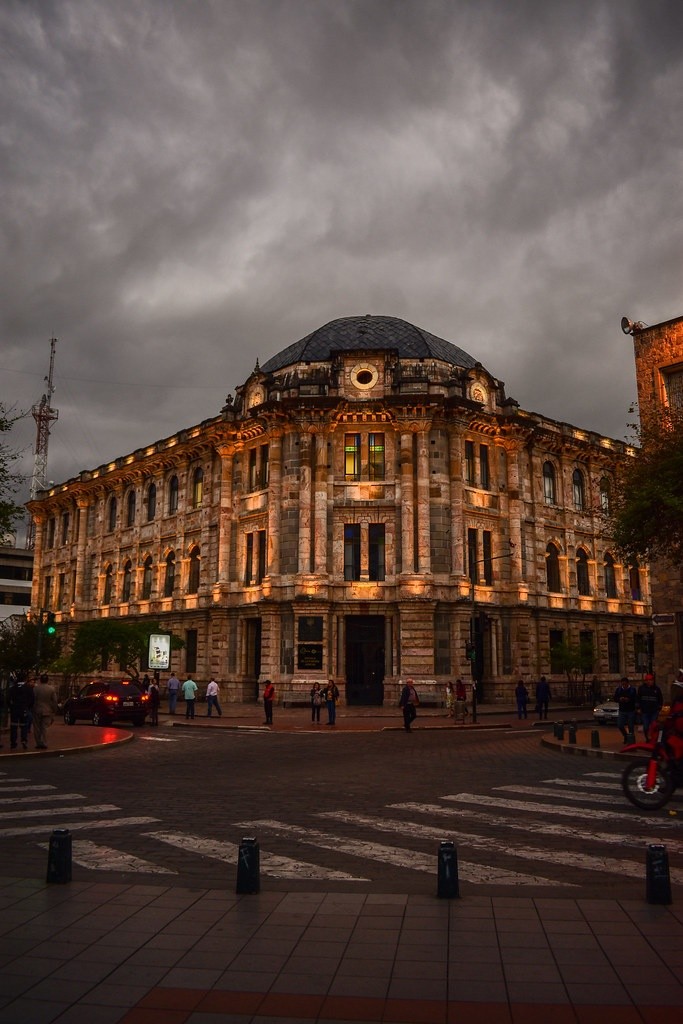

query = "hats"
645;674;654;680
264;680;271;684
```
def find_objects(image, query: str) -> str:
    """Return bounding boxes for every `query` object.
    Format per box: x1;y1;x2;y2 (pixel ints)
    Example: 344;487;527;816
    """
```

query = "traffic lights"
47;611;57;637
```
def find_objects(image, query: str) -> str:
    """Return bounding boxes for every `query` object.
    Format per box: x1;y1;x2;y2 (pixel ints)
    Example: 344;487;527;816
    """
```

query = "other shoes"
35;744;47;749
318;722;321;725
12;742;18;750
270;721;273;724
22;742;28;749
326;722;334;725
312;722;315;724
263;721;268;724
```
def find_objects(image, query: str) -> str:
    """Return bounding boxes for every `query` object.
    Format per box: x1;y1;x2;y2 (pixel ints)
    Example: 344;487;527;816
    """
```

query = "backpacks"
313;690;323;705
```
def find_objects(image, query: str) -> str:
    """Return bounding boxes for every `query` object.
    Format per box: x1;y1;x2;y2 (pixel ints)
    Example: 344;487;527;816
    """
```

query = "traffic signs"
651;614;676;627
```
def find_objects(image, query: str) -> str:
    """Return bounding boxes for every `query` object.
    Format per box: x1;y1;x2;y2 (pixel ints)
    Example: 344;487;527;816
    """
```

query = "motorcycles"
620;703;683;812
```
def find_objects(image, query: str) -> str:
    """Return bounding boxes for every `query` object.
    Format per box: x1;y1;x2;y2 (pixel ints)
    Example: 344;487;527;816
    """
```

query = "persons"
7;673;34;751
325;680;339;725
444;681;454;718
591;675;603;706
536;677;552;720
310;682;324;725
638;674;664;742
515;680;528;720
455;679;468;716
150;687;160;727
205;677;222;716
148;678;159;694
29;678;34;687
182;674;201;719
142;674;150;691
32;674;57;749
166;673;180;712
399;679;419;733
263;680;274;725
613;678;638;744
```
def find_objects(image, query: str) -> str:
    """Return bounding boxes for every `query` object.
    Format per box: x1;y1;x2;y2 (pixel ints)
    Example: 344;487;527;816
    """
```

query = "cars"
63;679;151;728
593;699;640;727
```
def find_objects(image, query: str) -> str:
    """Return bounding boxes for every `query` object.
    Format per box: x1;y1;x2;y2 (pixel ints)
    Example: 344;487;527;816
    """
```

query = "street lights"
471;547;515;724
